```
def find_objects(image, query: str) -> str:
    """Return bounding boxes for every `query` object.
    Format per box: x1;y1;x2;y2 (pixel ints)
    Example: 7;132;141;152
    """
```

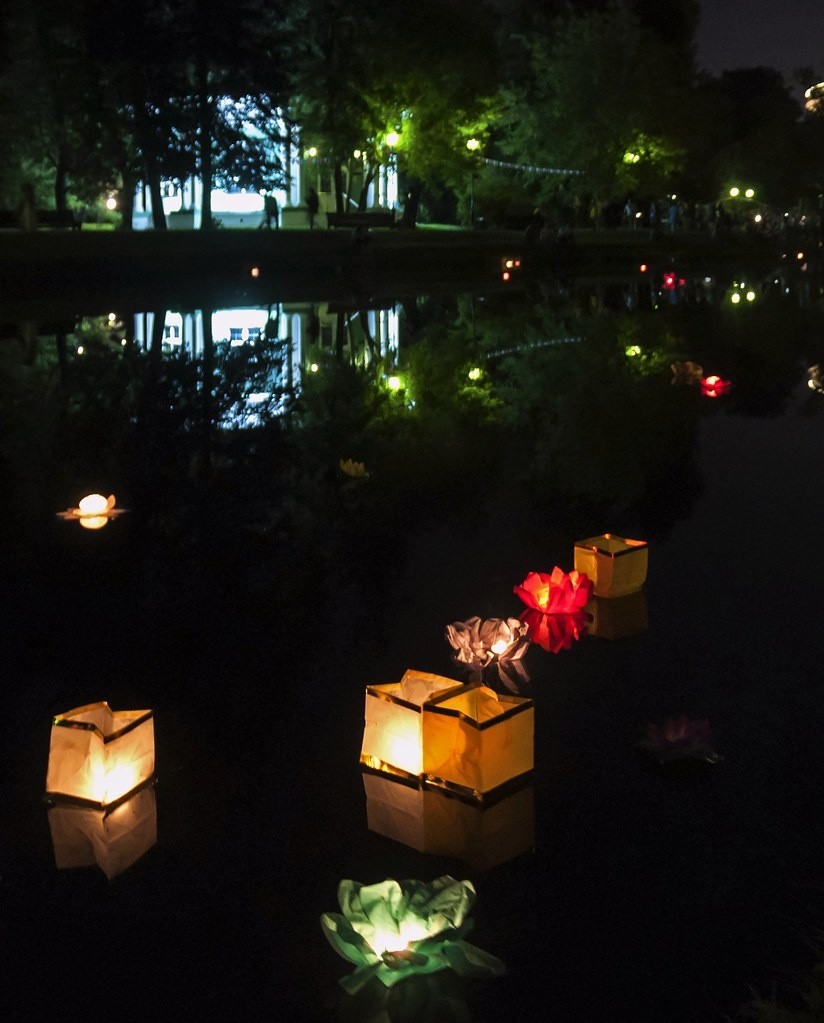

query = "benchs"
33;209;82;230
325;211;395;230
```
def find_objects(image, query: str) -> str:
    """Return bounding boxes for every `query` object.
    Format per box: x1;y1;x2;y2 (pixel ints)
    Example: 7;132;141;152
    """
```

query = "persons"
68;205;92;232
649;201;656;227
623;199;634;232
522;207;557;245
666;194;728;236
762;207;823;241
586;198;599;231
264;194;279;232
398;190;413;221
305;186;320;230
